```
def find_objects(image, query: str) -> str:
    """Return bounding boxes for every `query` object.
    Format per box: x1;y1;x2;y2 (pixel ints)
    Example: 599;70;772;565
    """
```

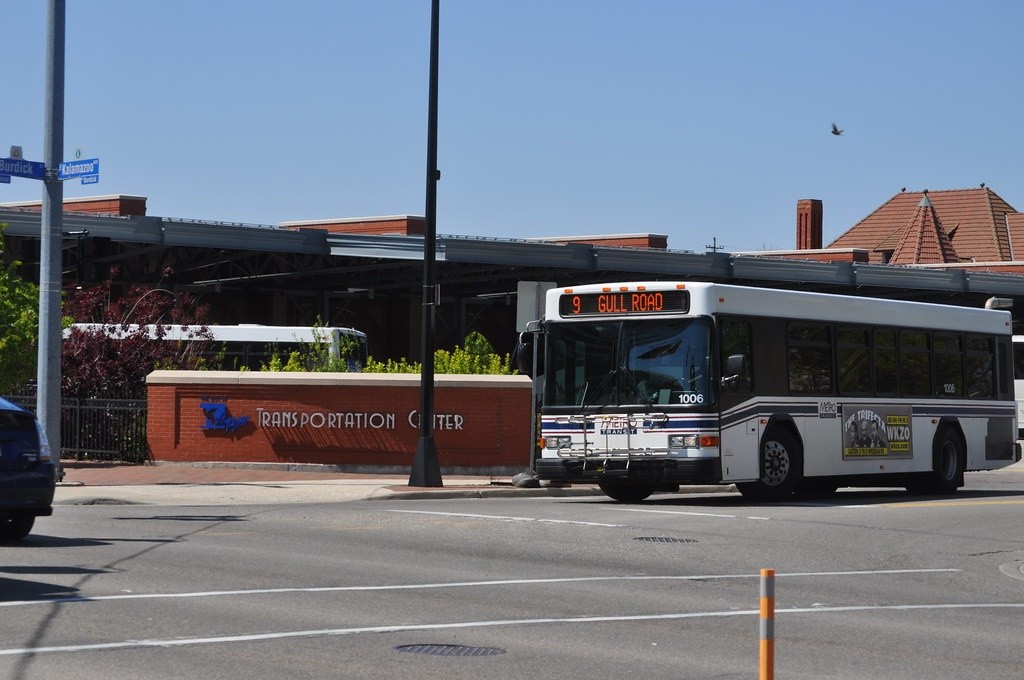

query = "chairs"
726;354;750;398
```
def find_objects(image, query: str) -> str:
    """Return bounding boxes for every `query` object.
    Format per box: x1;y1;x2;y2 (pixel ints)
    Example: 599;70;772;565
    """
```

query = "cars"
0;396;57;547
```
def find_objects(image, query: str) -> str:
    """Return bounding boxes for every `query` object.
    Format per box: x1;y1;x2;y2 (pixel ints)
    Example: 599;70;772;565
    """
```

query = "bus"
63;321;370;382
516;280;1023;507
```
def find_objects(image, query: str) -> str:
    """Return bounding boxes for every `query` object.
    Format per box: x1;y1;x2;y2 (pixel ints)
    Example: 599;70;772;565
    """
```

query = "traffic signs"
57;158;100;186
0;158;46;183
